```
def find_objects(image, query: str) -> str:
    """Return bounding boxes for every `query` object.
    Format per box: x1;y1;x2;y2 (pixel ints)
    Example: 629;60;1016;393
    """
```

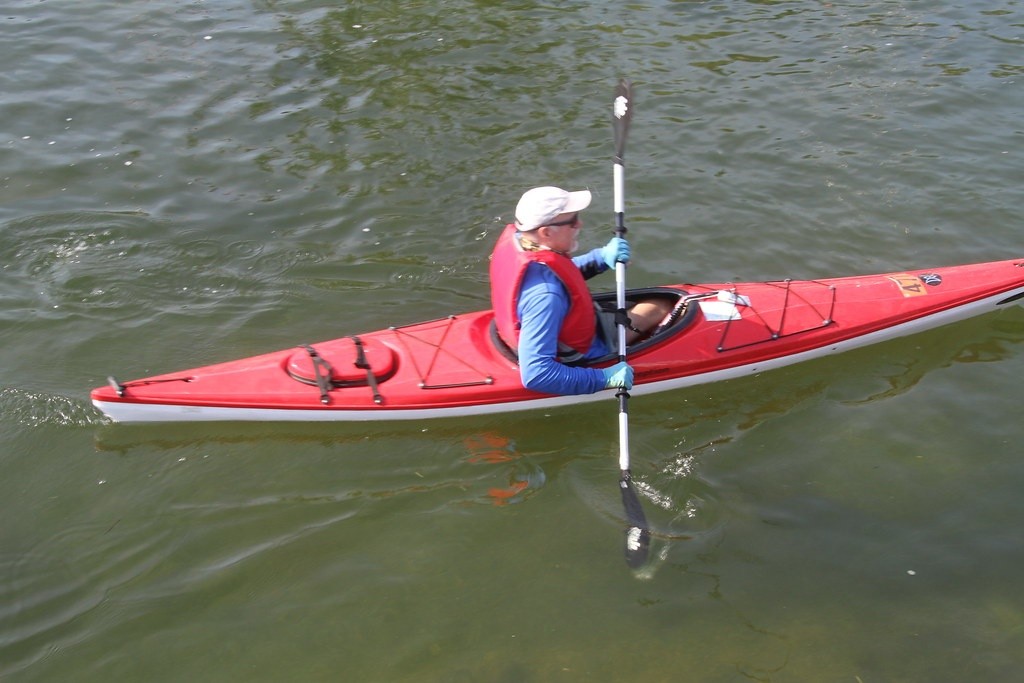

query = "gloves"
602;238;630;271
602;362;634;390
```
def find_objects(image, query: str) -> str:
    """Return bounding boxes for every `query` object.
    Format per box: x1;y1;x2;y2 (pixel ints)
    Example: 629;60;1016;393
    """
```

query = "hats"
514;186;592;231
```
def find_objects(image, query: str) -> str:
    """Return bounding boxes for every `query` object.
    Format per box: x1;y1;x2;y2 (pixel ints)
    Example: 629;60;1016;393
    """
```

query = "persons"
490;186;677;395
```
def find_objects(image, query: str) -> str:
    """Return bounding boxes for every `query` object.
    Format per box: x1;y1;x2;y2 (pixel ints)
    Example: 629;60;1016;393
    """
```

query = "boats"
89;251;1022;424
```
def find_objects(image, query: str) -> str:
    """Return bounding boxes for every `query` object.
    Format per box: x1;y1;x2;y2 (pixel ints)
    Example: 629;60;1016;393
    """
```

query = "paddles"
610;75;650;529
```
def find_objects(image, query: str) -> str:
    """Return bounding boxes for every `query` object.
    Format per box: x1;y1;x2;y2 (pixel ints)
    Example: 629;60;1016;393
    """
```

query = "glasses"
534;212;578;230
